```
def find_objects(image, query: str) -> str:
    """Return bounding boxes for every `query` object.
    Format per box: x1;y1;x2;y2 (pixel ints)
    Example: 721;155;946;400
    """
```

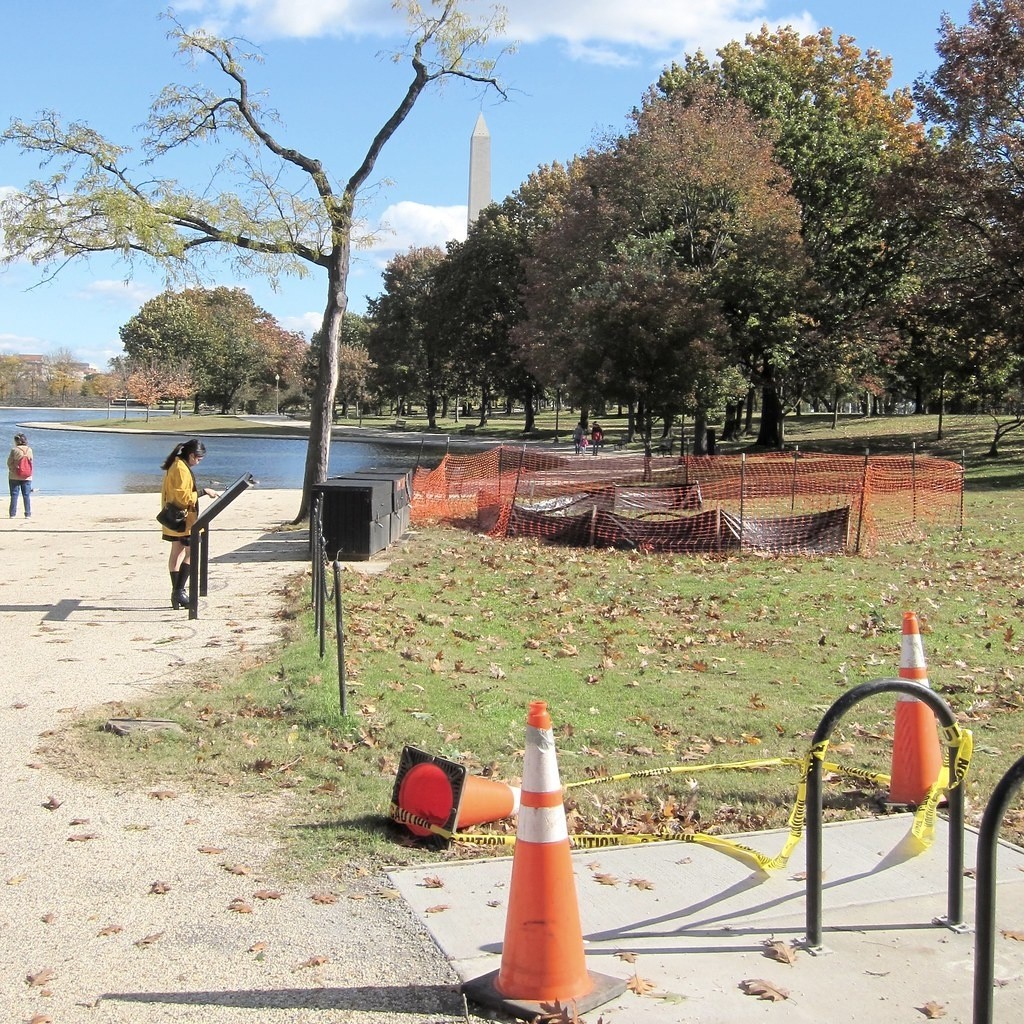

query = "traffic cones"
458;700;628;1024
384;743;522;851
877;611;950;814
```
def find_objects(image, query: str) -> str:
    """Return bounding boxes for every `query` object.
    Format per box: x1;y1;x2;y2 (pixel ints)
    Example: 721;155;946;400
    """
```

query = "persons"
7;433;33;519
161;438;220;610
590;421;603;456
573;422;589;456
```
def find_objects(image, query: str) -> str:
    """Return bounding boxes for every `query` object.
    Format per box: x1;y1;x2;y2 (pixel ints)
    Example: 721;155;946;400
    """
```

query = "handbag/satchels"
156;501;187;533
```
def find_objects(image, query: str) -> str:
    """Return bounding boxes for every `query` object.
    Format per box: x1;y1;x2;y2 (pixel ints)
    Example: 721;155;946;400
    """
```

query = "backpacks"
592;431;603;442
581;439;588;447
13;446;33;478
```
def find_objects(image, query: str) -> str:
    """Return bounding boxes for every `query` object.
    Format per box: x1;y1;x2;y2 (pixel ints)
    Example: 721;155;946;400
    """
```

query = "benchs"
409;410;417;418
391;421;406;431
587;432;603;448
613;434;628;450
651;437;675;457
459;423;475;435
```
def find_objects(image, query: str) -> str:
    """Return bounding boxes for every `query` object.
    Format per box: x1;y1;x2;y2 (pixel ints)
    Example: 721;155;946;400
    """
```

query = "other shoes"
25;517;30;519
10;516;15;519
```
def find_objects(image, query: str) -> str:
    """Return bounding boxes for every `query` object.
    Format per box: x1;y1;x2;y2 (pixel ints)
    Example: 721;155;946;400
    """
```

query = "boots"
171;562;190;610
170;571;184;607
